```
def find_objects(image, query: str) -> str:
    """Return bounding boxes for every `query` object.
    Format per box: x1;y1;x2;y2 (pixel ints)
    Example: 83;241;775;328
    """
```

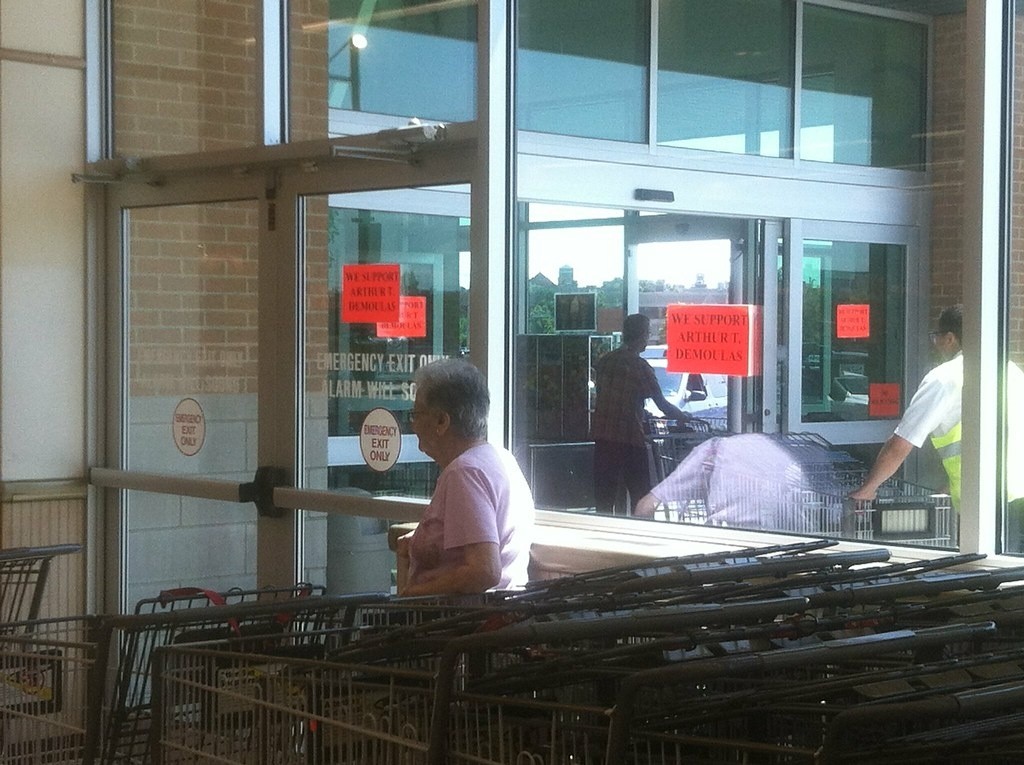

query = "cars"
824;372;875;419
641;356;727;434
639;344;669;360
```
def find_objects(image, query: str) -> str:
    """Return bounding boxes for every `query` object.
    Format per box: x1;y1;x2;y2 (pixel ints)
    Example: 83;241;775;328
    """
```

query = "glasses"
927;330;946;345
407;409;432;423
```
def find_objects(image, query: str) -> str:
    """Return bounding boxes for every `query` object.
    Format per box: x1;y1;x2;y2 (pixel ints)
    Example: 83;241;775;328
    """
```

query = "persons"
634;432;842;533
399;358;535;598
850;303;1024;555
587;314;693;517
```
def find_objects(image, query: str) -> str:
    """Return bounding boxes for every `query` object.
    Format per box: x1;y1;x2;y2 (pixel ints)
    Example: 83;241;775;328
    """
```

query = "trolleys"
1;416;1024;764
